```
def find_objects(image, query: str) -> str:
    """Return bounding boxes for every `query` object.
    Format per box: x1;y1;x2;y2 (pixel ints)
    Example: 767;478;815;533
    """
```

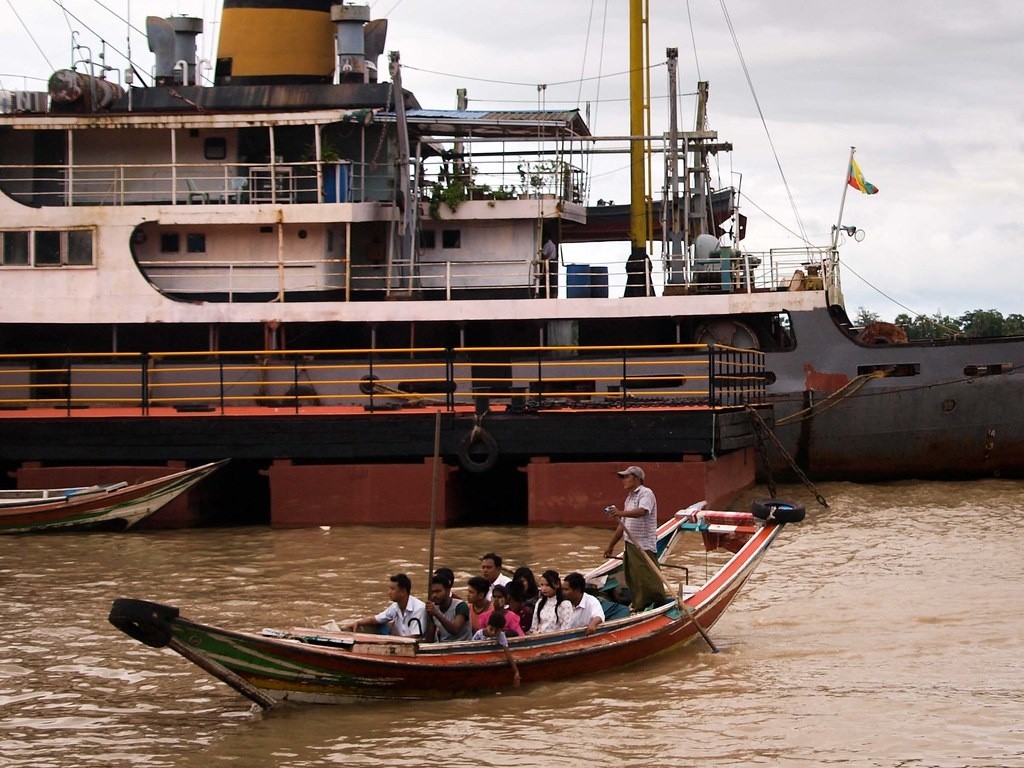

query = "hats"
616;466;645;481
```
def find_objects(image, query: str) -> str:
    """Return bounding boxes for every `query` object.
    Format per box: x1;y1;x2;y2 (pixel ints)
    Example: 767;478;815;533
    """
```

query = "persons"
538;233;557;298
602;465;667;616
340;553;606;689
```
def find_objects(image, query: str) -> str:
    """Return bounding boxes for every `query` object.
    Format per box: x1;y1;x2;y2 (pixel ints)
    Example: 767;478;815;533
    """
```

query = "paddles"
602;500;724;657
415;406;446;643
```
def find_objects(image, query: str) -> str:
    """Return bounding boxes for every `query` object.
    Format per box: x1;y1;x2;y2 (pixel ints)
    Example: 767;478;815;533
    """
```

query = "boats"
106;494;809;713
1;0;1022;481
0;455;231;528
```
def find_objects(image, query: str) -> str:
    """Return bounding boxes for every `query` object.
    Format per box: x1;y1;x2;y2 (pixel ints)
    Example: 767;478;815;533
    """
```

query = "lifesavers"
458;427;500;472
750;494;808;526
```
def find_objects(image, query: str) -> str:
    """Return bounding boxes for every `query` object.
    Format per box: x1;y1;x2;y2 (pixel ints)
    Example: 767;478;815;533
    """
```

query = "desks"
249;167;297;205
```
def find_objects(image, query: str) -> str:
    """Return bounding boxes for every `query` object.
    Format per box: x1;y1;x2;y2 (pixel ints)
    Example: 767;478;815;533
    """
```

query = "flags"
848;158;879;195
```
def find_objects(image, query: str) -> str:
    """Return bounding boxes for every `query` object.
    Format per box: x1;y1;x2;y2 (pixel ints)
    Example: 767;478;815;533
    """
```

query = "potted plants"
437;149;561;201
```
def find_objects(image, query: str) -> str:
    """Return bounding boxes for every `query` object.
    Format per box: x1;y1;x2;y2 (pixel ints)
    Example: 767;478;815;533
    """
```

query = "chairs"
219;177;248;204
185;180;211;205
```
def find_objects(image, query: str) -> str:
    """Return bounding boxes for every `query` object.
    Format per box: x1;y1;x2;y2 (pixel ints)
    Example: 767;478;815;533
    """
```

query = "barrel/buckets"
322;162;353;203
590;267;608;297
567;265;590;298
